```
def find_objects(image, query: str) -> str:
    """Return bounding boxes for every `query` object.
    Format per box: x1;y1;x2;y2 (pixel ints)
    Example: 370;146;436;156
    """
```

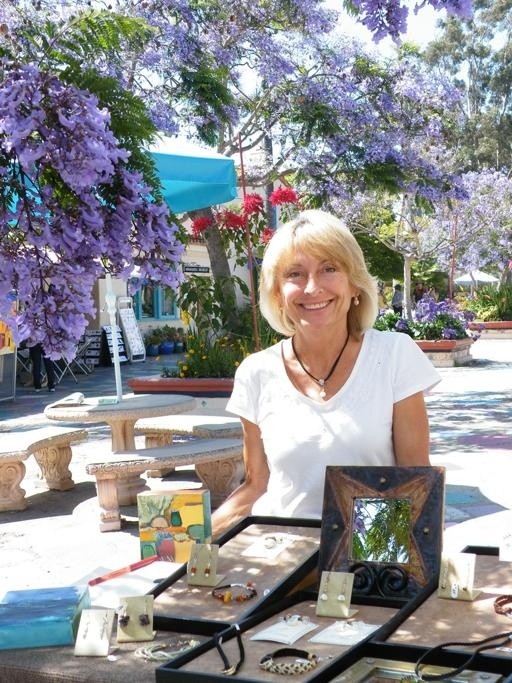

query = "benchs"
134;415;245;478
85;437;245;532
0;425;88;513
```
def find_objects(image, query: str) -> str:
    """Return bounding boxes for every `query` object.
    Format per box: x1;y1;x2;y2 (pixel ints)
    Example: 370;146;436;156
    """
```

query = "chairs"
16;337;93;385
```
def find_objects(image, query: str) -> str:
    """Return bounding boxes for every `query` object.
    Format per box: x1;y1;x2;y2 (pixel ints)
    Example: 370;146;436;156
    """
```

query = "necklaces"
291;330;350;397
401;632;512;683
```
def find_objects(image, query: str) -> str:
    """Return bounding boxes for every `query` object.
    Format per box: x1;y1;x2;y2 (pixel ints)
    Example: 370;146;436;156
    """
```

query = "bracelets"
260;647;319;675
134;639;200;662
214;622;244;675
211;583;258;603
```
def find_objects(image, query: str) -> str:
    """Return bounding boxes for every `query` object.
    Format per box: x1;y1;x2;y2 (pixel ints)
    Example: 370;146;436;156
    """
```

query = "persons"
30;343;55;393
210;210;443;540
412;283;428;303
391;284;403;316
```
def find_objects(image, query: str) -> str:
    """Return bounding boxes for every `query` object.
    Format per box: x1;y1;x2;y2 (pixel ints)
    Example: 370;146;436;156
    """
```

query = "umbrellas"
453;269;500;295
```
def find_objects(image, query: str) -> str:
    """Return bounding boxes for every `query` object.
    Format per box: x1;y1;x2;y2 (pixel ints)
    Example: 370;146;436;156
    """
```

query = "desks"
0;552;512;683
44;393;197;506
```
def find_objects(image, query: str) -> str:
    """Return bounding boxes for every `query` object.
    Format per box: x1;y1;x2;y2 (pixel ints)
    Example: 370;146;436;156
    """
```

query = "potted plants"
141;323;185;356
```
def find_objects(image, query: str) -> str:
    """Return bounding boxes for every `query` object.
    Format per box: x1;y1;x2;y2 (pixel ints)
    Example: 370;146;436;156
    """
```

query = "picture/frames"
316;466;445;599
327;656;504;683
